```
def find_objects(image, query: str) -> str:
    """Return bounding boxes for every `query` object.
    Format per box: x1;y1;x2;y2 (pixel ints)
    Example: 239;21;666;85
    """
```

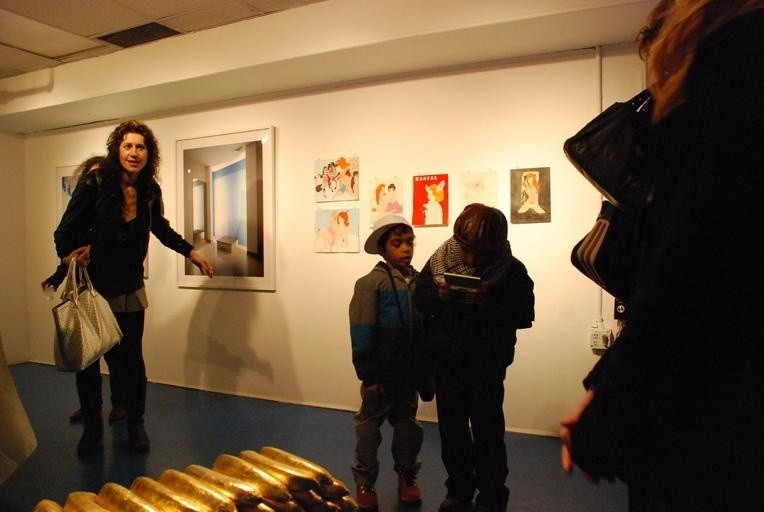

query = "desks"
216;236;239;253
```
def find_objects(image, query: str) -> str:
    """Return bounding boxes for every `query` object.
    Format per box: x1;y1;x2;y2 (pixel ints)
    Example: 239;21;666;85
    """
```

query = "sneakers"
77;415;104;460
439;489;473;511
398;478;422;502
126;416;149;453
473;502;507;512
109;405;127;422
70;407;102;421
356;484;378;509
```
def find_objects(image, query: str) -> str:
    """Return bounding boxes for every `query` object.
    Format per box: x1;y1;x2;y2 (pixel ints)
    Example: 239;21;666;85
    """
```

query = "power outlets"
589;329;612;350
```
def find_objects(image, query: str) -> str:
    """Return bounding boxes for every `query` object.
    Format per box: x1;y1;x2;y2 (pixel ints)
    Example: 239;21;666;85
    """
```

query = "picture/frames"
54;165;150;280
175;125;278;293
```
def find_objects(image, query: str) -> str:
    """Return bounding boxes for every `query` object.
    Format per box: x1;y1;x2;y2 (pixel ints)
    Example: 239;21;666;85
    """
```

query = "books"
444;272;483;307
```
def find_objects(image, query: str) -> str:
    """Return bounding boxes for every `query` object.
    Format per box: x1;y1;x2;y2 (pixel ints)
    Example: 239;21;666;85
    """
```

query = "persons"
348;214;443;511
421;203;535;510
39;157;130;423
557;1;763;511
52;119;214;465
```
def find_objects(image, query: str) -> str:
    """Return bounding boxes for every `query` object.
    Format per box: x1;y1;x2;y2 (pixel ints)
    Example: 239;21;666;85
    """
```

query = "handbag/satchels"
563;89;655;215
571;201;625;303
52;285;125;372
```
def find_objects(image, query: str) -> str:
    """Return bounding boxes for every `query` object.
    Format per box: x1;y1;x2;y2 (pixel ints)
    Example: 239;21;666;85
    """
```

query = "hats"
364;215;413;254
